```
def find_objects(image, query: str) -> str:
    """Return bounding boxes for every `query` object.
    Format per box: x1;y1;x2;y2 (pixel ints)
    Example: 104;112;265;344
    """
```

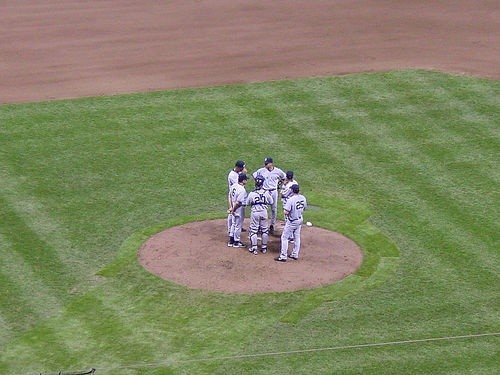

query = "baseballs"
306;221;312;227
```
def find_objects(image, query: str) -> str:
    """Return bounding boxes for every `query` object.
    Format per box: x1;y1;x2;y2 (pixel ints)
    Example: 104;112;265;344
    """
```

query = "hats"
286;170;293;179
289;184;299;190
238;173;250;180
234;160;246;168
254;176;264;185
263;157;273;163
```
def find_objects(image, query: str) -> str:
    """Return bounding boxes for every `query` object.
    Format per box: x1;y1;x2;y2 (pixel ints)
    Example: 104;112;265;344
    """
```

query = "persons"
274;184;308;262
227;173;250;248
228;161;247;234
281;170;300;244
252;157;286;234
246;180;272;255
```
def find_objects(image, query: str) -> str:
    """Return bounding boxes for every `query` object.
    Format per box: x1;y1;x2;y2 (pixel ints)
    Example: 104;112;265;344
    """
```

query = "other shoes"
287;238;294;243
248;248;258;255
268;225;276;232
242;228;246;232
228;241;246;248
273;257;286;262
260;248;267;253
288;254;297;260
